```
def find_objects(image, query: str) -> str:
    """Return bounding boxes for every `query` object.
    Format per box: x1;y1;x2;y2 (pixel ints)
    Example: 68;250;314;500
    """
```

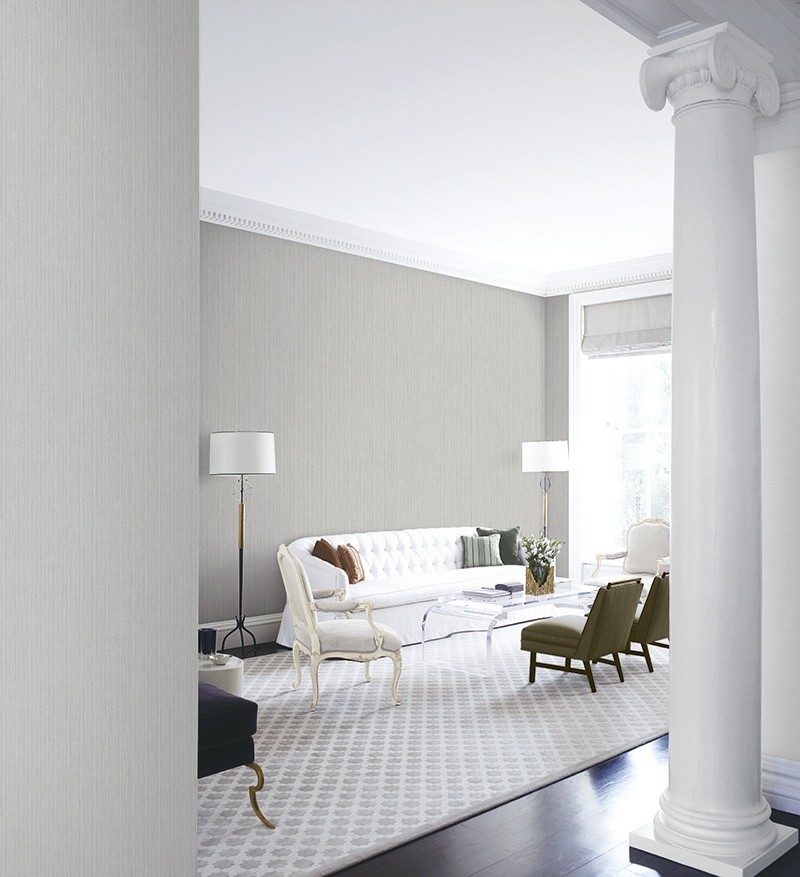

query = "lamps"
520;441;568;539
209;430;276;656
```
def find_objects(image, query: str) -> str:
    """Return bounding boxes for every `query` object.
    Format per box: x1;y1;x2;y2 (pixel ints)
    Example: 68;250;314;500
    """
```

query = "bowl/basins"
209;655;232;665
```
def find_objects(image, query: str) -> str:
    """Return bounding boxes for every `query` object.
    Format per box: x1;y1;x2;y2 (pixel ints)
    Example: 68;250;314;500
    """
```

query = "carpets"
197;608;670;876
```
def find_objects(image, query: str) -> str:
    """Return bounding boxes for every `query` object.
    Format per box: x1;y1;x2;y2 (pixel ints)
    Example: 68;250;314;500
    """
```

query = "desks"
198;653;245;695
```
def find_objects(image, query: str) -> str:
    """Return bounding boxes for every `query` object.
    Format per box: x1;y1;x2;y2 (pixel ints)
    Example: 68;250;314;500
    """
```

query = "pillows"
475;526;523;565
336;543;364;583
313;538;342;569
460;532;505;568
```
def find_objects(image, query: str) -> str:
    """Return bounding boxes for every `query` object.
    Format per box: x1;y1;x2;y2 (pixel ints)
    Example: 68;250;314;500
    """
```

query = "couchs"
276;526;531;656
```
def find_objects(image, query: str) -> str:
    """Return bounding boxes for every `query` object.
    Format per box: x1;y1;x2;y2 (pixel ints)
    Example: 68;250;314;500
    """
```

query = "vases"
524;566;556;596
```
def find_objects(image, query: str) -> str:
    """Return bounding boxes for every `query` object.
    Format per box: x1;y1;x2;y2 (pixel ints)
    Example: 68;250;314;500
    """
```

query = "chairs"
589;517;670;603
520;578;643;692
587;571;669;673
276;543;402;711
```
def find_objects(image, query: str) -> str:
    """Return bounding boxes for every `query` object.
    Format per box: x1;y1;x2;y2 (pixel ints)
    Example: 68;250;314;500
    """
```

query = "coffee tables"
418;581;604;680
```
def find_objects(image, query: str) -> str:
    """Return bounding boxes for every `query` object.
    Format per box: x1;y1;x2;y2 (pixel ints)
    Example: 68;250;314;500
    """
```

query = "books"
463;589;508;597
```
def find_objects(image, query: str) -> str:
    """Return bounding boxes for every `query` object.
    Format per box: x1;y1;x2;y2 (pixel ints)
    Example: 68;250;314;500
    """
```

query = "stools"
198;681;276;828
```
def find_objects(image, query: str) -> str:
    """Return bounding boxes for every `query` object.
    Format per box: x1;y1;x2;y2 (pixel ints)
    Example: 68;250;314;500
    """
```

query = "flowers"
520;530;566;568
